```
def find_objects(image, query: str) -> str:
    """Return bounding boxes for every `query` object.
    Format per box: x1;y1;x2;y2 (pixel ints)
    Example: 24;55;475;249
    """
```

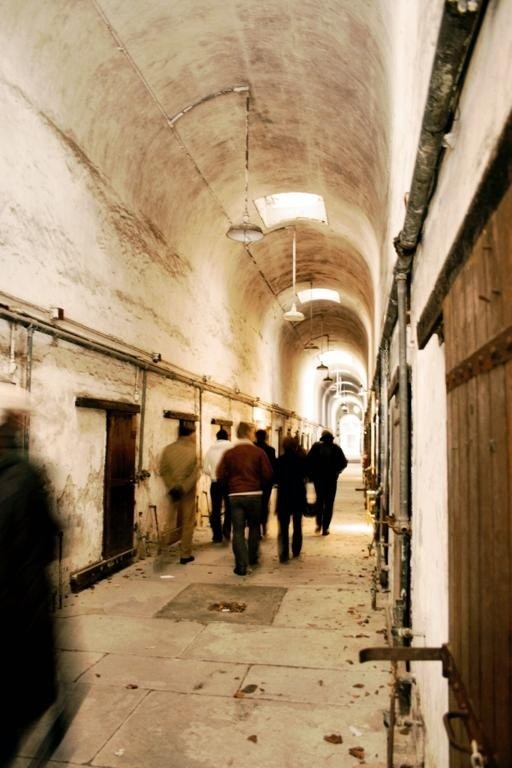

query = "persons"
1;408;86;766
159;422;348;576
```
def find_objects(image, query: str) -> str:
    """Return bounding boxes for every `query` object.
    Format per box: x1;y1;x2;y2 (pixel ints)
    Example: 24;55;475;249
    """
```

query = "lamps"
303;281;320;352
226;93;264;242
316;316;329;370
336;372;348;415
324;371;333;382
283;225;305;322
